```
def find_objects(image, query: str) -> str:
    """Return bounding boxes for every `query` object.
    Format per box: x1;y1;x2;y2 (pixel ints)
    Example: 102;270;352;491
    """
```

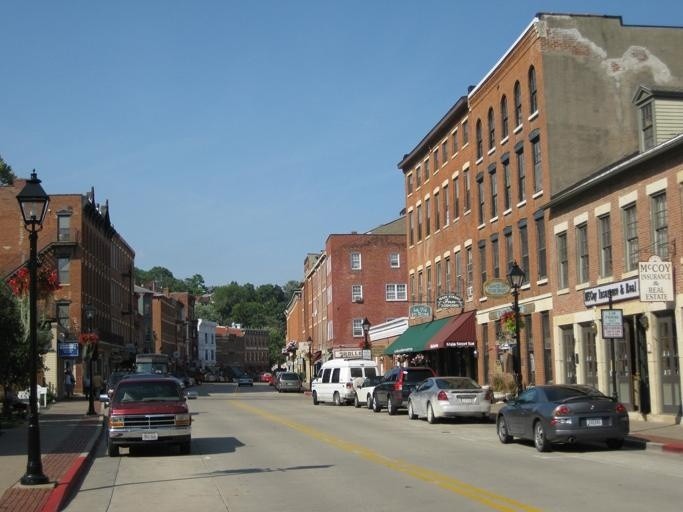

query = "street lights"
175;319;186;376
505;258;527;398
306;335;314;391
15;165;54;487
361;316;371;349
83;309;96;417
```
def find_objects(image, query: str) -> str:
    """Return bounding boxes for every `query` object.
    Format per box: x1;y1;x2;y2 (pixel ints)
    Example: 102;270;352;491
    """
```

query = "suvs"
96;369;203;458
370;366;438;416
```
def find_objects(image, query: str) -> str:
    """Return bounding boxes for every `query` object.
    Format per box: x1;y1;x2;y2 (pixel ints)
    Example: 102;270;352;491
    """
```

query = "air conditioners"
356;296;361;301
467;287;473;297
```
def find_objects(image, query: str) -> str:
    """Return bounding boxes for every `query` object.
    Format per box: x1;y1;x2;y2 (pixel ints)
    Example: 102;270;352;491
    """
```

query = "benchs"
2;398;32;420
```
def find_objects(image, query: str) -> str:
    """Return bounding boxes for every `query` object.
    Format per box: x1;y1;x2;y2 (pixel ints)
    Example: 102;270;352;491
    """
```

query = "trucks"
308;355;379;406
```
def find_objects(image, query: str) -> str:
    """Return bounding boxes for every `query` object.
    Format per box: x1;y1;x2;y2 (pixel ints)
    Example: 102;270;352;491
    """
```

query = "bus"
132;352;171;374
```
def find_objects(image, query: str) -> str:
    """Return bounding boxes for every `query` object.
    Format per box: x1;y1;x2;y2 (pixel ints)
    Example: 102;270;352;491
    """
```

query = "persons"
63;367;104;400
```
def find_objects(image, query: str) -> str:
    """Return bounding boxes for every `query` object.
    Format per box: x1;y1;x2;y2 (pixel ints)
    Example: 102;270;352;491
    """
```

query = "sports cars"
493;380;631;454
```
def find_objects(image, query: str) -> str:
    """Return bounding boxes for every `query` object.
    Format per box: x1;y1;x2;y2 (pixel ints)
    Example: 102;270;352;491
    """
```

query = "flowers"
357;340;375;350
499;310;522;327
78;332;100;345
7;262;63;301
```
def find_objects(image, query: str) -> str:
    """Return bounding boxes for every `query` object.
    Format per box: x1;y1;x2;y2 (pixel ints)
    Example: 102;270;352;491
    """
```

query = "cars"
202;371;219;382
353;375;383;410
258;371;303;393
238;371;253;387
406;375;492;424
